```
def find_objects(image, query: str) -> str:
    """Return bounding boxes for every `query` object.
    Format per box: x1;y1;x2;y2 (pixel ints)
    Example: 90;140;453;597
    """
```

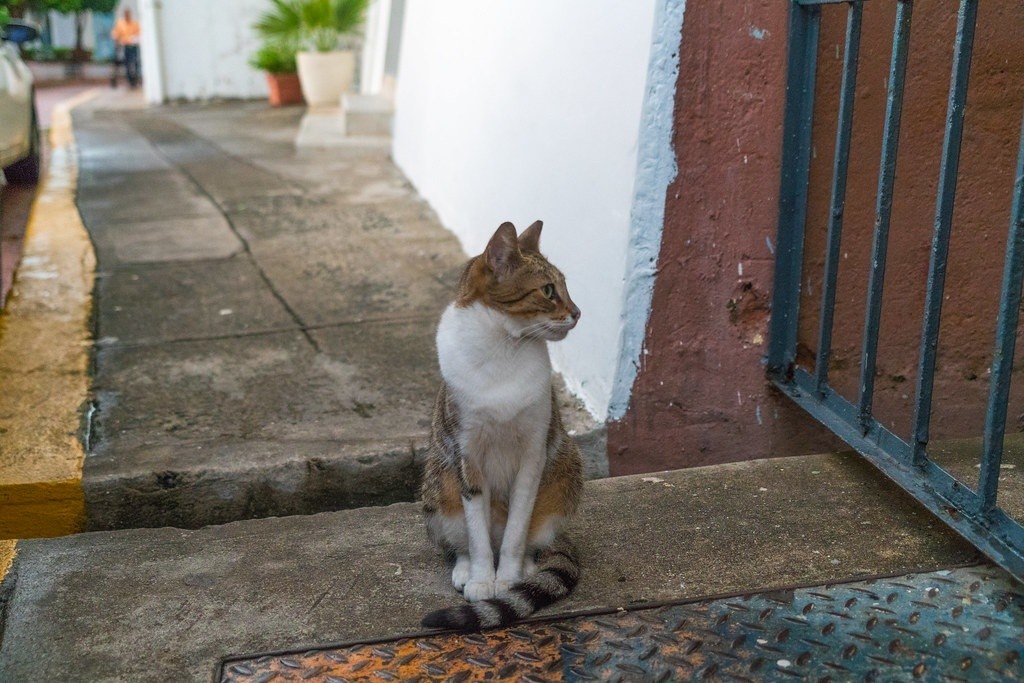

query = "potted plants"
248;0;378;108
246;43;310;107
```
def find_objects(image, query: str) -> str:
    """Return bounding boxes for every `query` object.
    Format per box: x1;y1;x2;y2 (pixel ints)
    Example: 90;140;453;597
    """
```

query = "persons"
111;8;140;90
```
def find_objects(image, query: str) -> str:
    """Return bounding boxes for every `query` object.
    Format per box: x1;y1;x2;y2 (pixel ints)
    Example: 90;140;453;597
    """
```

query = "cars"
1;17;42;186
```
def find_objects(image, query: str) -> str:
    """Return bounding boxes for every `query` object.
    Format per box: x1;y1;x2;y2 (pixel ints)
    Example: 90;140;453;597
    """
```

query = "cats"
423;221;584;633
